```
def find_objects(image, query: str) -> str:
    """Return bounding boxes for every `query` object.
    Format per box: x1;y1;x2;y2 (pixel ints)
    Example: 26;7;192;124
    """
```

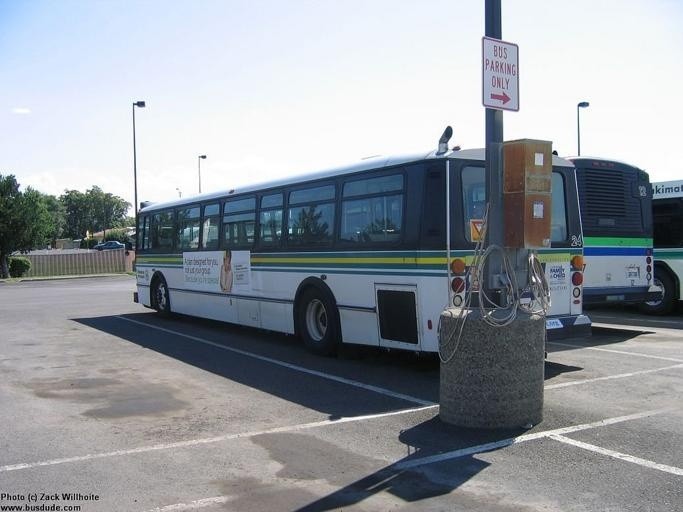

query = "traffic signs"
480;34;520;113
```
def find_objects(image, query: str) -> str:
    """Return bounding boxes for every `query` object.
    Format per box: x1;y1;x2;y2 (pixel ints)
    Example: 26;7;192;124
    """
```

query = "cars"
93;240;126;251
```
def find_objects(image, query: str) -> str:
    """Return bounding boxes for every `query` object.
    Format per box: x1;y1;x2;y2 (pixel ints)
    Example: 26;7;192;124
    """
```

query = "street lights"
577;101;590;157
132;100;146;221
198;155;207;194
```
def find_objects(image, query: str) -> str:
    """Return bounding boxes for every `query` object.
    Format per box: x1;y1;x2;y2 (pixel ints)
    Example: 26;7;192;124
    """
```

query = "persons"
219;250;233;292
